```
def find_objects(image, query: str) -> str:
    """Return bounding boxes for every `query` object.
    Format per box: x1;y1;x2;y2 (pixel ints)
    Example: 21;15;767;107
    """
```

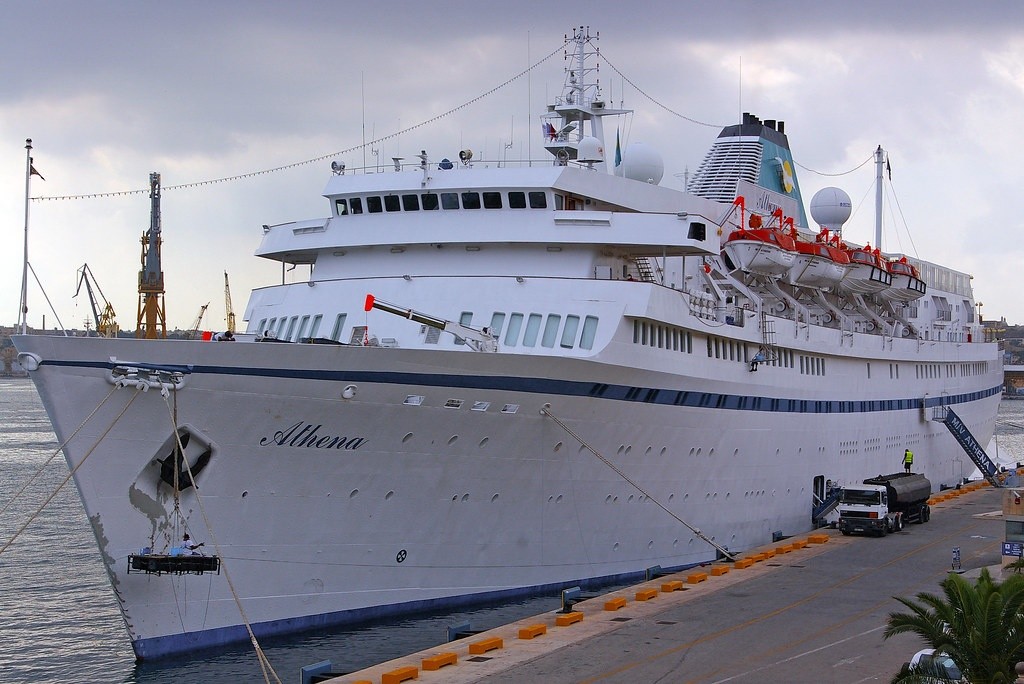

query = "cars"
907;650;968;684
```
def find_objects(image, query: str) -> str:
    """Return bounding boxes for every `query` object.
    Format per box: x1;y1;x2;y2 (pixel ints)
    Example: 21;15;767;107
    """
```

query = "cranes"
184;300;211;341
223;269;236;335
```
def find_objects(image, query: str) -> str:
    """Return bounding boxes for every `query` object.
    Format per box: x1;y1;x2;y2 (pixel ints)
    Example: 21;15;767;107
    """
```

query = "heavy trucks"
836;472;931;538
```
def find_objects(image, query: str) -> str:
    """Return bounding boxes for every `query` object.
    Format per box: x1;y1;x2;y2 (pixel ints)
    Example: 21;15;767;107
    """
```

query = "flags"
543;122;557;139
614;129;621;167
30;165;45;180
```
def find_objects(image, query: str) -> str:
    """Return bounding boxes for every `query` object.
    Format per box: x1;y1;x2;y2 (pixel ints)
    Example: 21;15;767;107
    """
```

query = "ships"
5;23;1007;665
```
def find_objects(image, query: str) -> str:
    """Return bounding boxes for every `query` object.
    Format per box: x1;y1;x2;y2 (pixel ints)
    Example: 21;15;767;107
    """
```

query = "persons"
902;449;913;474
749;344;763;372
181;534;205;556
214;331;236;341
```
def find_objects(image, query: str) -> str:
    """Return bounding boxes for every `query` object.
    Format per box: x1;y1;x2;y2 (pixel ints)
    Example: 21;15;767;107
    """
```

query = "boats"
875;257;927;302
782;240;850;291
724;228;801;277
838;249;892;296
